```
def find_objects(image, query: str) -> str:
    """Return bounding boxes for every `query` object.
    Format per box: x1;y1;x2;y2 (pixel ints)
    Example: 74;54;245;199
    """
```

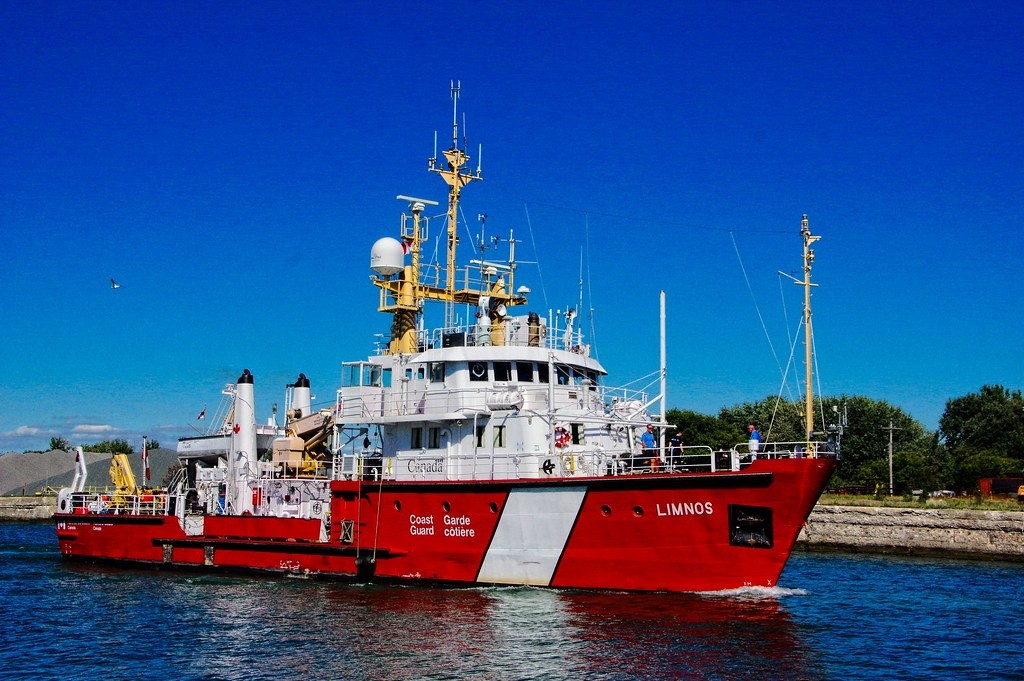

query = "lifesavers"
555;427;571;448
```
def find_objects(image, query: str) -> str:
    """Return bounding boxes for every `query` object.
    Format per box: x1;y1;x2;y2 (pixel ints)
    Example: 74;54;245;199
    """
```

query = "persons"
641;423;656;471
747;424;762;452
667;429;684;472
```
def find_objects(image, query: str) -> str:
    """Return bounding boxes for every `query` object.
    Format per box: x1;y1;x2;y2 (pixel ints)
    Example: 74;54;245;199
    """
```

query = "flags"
198;409;204;420
401;231;419;255
141;440;151;480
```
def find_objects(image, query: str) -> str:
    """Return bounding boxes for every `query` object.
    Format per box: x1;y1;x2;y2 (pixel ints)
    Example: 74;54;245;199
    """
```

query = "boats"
56;78;840;595
